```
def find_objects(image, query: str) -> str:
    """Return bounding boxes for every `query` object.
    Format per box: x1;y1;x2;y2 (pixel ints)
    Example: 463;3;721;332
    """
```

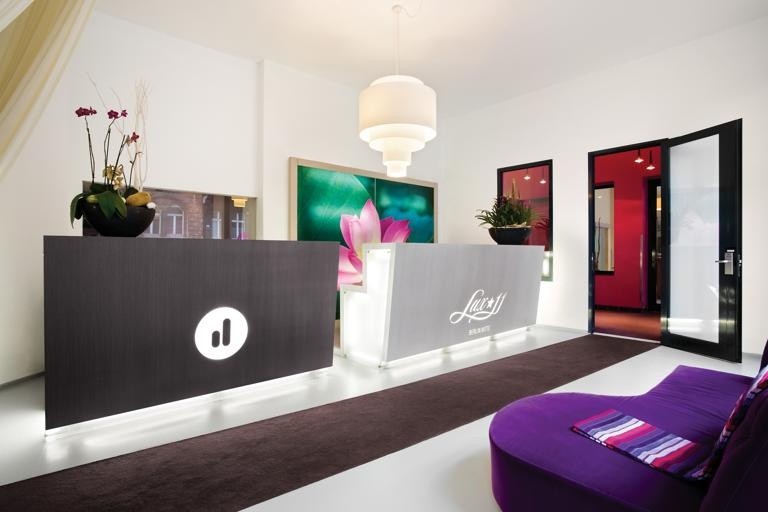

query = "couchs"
488;338;766;511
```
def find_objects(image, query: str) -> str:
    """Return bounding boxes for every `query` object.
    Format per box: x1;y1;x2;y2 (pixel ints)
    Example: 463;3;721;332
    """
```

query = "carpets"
1;334;662;512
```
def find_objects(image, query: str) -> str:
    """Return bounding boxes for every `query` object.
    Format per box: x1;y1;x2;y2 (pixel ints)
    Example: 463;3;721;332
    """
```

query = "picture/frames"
287;155;438;321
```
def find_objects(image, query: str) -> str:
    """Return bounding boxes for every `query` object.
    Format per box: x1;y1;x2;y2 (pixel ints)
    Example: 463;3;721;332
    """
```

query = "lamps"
634;149;656;171
524;165;547;185
355;6;442;178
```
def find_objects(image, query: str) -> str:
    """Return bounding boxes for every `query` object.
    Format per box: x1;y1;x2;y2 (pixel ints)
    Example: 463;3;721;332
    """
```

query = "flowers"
474;183;548;229
60;104;154;228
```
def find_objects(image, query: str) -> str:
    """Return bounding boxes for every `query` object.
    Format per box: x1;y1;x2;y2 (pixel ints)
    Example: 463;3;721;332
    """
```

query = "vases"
83;195;155;238
487;224;531;243
